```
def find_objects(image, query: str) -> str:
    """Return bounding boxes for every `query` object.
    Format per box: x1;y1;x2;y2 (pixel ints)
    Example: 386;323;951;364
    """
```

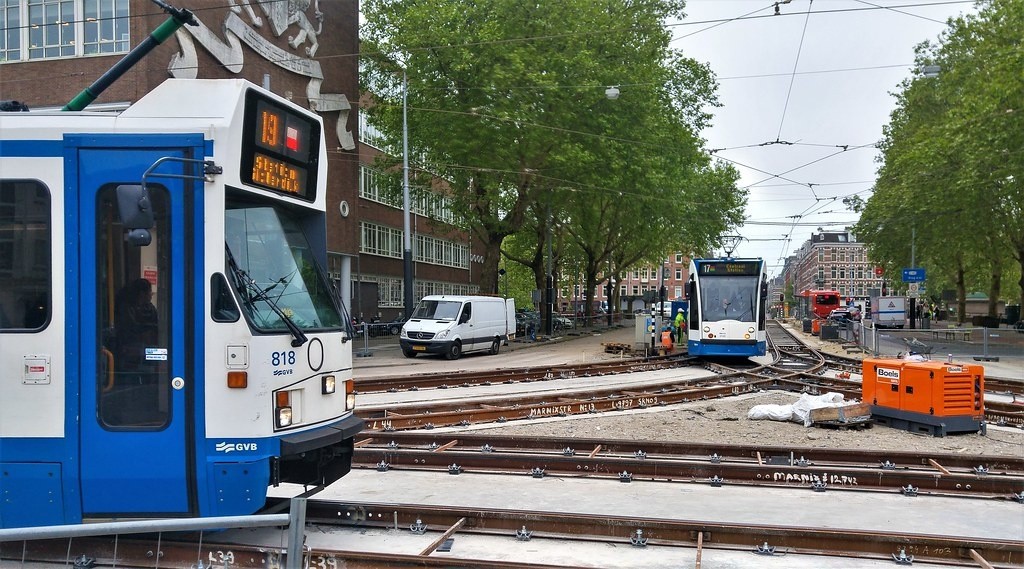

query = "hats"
678;308;684;312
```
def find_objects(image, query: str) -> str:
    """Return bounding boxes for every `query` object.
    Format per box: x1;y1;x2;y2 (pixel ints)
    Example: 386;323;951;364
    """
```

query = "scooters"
351;311;404;338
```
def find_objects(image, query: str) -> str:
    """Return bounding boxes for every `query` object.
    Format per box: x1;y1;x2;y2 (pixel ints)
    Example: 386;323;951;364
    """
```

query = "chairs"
945;323;972;340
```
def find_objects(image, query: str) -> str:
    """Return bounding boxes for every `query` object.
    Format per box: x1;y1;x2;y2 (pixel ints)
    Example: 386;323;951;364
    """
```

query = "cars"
847;306;862;323
655;301;671;318
515;300;616;333
827;308;852;327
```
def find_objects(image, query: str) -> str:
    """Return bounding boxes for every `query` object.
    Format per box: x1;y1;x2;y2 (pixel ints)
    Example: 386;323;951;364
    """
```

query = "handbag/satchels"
924;313;930;318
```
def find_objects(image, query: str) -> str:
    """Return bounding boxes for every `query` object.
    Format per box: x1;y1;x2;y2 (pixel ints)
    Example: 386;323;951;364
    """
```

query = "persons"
352;317;357;325
934;307;939;323
121;278;158;333
718;299;733;314
660;327;675;347
674;307;684;346
916;305;921;321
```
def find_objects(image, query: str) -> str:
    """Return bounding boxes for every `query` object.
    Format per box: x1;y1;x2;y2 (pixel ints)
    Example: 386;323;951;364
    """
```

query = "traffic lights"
779;293;785;302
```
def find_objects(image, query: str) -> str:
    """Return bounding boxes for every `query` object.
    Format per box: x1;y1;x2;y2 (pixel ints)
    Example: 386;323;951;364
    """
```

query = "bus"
800;289;841;319
0;1;366;532
683;235;769;361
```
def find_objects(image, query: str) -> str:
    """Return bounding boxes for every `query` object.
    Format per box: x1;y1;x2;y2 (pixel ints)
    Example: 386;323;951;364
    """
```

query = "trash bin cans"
922;317;930;329
1006;305;1020;325
811;320;827;336
803;318;811;333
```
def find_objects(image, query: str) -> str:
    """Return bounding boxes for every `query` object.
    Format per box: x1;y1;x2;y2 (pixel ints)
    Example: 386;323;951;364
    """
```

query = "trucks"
870;296;906;330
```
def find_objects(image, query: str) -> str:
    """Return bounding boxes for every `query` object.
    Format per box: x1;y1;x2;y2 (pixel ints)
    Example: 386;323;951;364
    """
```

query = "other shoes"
936;322;937;324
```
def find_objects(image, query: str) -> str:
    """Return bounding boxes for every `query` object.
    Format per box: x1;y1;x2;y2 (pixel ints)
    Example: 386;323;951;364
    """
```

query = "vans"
399;294;516;361
